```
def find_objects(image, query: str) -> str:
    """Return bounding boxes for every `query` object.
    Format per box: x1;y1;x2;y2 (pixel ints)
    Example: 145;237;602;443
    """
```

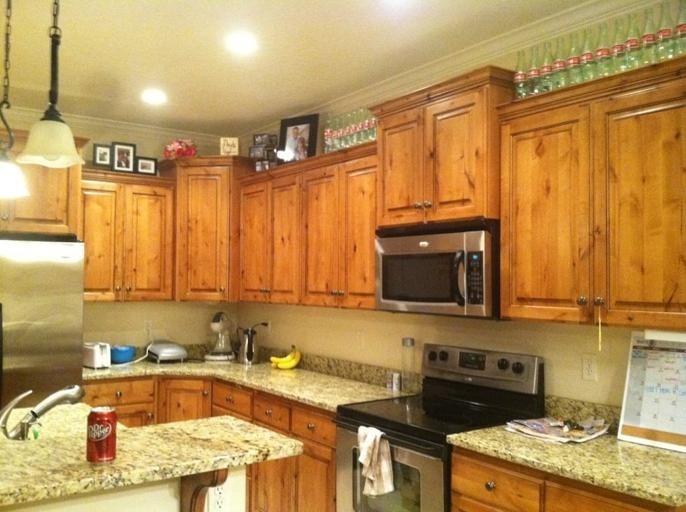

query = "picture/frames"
278;113;319;166
113;142;136;173
136;156;157;176
93;142;112;168
219;136;239;156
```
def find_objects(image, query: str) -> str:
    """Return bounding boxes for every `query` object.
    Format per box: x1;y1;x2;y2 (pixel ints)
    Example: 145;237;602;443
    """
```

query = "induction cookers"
337;392;517;444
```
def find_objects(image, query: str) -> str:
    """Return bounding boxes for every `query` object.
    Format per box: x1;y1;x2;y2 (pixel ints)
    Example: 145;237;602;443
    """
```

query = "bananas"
270;345;301;370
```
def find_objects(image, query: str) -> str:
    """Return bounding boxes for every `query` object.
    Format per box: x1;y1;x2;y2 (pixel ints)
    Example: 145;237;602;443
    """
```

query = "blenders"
204;310;236;361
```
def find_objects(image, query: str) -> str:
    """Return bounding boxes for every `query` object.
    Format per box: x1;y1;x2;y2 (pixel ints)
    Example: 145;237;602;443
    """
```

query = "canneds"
87;407;116;464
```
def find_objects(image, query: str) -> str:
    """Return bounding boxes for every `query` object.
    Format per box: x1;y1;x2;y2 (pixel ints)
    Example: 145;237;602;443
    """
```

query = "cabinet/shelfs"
211;377;253;511
368;65;512;229
251;387;337;511
494;54;686;332
80;375;158;427
159;156;256;304
450;445;544;512
0;129;91;241
82;167;176;303
300;141;376;310
237;161;299;304
159;377;212;423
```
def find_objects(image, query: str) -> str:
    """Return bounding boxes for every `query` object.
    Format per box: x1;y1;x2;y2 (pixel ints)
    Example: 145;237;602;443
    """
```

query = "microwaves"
374;227;500;321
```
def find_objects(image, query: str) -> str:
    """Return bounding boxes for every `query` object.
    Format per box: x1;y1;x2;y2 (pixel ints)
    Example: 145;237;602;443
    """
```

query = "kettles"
238;327;259;365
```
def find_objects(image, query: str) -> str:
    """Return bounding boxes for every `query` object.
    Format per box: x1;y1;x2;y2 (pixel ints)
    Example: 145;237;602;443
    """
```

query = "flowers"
163;137;198;160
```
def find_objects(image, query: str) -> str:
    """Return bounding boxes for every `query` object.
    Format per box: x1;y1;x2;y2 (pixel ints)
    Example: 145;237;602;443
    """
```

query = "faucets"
0;385;86;439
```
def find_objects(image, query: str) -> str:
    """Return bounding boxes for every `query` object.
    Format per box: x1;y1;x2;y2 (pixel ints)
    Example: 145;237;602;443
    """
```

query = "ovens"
332;417;452;512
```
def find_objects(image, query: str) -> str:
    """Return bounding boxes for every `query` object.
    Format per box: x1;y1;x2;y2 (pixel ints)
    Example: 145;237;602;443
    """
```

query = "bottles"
401;337;417;394
514;0;686;100
323;108;378;151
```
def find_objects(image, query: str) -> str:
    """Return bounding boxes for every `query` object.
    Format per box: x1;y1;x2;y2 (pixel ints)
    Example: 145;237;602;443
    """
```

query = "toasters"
83;342;111;370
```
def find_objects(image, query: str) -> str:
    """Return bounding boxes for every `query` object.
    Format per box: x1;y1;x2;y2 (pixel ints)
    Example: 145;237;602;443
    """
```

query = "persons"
294;136;308;161
283;126;301;162
99;152;107;162
116;151;130;169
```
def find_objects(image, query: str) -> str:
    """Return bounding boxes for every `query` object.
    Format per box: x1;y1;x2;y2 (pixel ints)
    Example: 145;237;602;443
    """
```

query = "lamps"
0;1;33;199
15;1;86;168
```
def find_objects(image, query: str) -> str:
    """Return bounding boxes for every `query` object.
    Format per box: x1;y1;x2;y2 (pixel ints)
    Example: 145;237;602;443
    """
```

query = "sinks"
0;403;128;439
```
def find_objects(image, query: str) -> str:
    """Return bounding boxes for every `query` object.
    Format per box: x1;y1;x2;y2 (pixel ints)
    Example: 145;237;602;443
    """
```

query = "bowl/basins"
110;345;136;363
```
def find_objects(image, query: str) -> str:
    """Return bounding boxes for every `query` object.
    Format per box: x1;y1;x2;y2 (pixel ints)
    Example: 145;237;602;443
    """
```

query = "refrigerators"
0;239;86;411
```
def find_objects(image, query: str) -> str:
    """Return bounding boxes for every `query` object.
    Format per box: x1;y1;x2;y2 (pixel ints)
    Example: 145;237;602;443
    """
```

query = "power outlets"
259;320;272;332
